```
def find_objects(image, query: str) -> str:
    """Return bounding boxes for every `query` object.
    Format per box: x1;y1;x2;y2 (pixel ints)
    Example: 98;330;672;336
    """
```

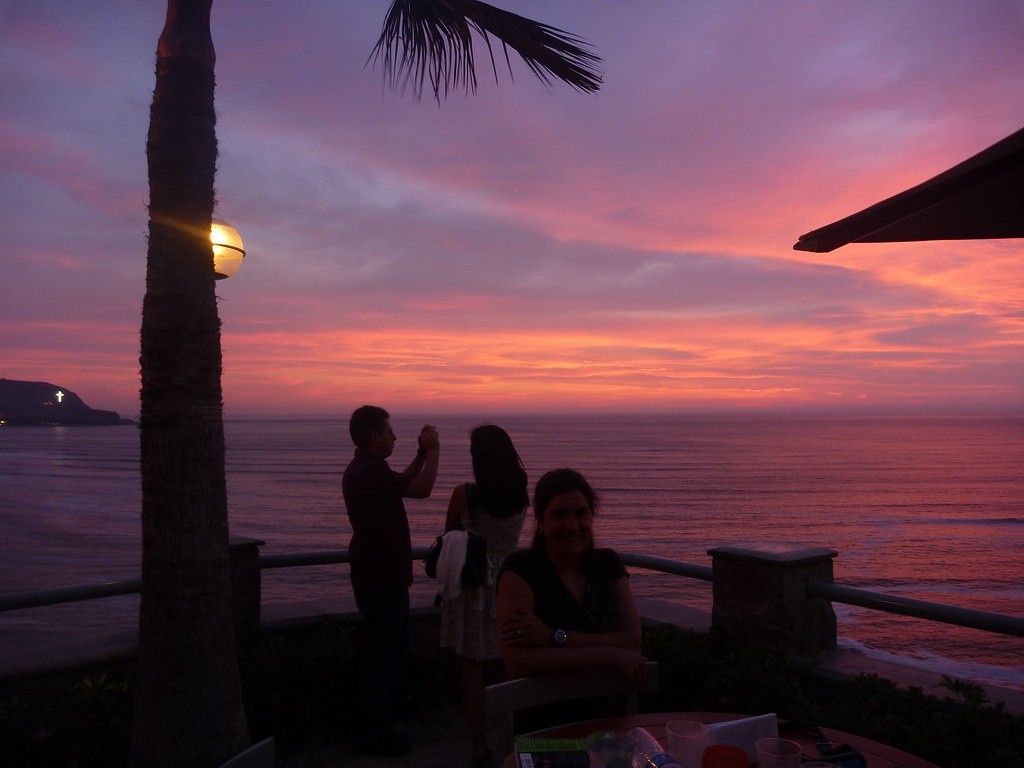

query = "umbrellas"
791;126;1024;255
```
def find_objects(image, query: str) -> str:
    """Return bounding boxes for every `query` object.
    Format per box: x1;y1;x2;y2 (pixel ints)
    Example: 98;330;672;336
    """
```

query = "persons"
338;405;440;754
494;467;656;738
436;424;531;767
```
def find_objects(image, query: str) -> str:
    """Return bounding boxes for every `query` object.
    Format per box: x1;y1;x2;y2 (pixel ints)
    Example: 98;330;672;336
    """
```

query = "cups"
700;744;750;768
799;762;842;768
666;719;709;768
754;737;803;768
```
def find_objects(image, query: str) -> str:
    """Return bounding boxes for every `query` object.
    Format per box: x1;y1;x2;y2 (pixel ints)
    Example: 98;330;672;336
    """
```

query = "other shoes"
354;740;410;758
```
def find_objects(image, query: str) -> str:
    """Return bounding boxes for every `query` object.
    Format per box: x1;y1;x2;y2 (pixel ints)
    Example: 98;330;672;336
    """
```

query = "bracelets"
417;448;430;458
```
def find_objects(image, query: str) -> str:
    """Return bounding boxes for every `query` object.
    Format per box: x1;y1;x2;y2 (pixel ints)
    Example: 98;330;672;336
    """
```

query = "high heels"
472;747;494;768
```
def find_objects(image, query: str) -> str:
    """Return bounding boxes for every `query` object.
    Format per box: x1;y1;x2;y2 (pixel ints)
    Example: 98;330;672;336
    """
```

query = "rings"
517;629;522;636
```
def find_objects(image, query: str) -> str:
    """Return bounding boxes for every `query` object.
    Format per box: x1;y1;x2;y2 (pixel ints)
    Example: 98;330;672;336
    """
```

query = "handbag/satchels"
425;531;485;585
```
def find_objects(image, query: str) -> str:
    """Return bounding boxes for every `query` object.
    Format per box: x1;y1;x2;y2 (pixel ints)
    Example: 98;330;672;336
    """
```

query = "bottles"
622;727;684;768
589;738;633;768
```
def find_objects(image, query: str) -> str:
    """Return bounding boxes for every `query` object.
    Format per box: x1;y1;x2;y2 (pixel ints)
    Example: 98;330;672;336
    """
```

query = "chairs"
220;737;277;768
486;662;634;768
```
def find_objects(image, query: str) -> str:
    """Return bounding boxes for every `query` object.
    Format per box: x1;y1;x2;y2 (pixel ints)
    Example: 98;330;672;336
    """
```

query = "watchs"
552;628;567;649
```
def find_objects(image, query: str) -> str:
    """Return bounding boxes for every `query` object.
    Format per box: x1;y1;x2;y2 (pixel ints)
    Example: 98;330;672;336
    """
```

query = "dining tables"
502;710;940;768
